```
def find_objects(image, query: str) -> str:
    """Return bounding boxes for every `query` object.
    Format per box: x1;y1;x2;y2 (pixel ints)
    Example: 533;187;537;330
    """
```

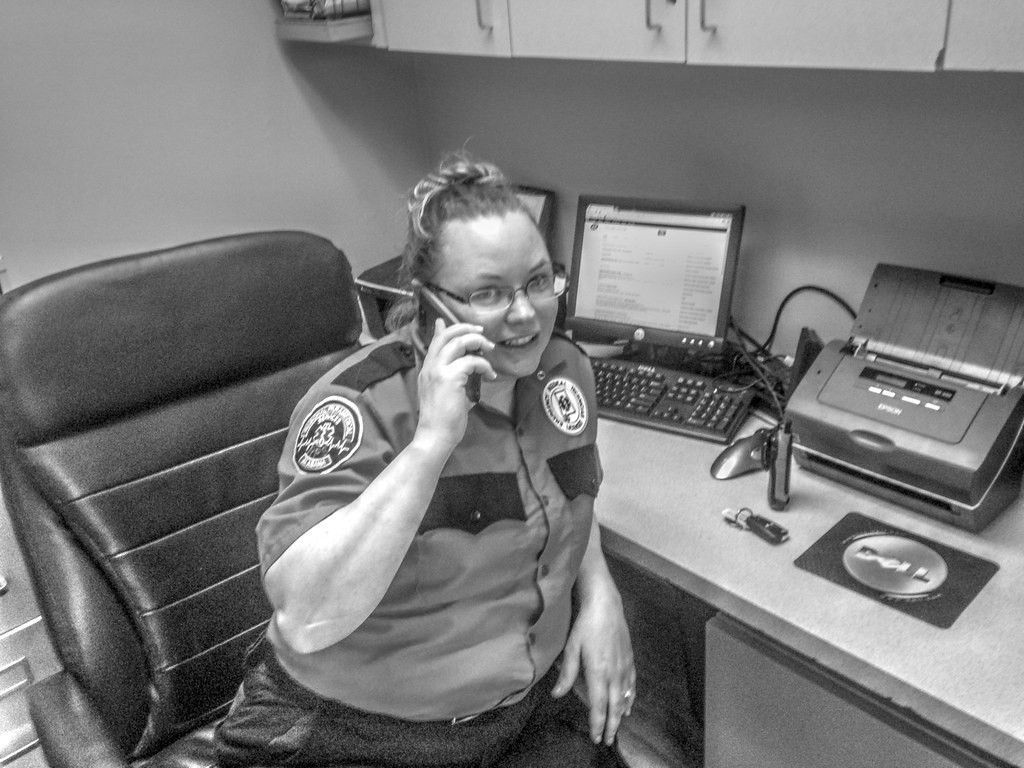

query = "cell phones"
417;287;484;401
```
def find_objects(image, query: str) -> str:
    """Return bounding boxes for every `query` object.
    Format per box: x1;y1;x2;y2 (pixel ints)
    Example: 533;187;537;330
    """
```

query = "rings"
623;689;633;698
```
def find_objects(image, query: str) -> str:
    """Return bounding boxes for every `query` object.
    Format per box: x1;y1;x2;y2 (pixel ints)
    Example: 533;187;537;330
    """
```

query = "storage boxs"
355;254;416;339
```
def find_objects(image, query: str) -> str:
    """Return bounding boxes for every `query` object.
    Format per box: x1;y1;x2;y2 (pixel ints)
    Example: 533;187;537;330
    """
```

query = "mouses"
709;435;775;480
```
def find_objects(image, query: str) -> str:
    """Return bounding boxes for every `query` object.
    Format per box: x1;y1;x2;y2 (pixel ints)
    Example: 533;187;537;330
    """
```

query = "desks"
566;326;1024;768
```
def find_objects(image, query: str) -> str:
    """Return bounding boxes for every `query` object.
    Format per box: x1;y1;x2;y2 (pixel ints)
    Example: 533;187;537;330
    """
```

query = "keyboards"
587;357;758;443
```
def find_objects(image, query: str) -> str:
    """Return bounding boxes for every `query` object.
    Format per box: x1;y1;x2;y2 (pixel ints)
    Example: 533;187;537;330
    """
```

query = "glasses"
423;262;569;312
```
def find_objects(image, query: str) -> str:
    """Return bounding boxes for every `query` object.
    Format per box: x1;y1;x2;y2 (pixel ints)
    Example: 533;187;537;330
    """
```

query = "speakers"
784;327;824;403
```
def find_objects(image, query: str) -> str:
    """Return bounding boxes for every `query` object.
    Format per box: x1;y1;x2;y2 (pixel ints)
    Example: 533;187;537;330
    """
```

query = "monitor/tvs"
563;195;745;372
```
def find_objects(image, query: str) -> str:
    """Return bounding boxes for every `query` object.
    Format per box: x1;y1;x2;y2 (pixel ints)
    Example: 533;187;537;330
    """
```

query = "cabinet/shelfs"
275;0;1024;72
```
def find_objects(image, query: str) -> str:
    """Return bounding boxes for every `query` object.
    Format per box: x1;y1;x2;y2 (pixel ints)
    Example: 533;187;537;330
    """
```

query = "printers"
781;262;1024;534
354;184;569;339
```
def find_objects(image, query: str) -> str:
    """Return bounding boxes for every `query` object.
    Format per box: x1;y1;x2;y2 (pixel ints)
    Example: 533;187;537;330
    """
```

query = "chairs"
0;231;629;768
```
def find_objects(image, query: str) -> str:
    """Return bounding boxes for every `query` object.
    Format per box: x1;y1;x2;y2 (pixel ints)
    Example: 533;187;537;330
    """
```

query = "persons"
208;155;639;768
